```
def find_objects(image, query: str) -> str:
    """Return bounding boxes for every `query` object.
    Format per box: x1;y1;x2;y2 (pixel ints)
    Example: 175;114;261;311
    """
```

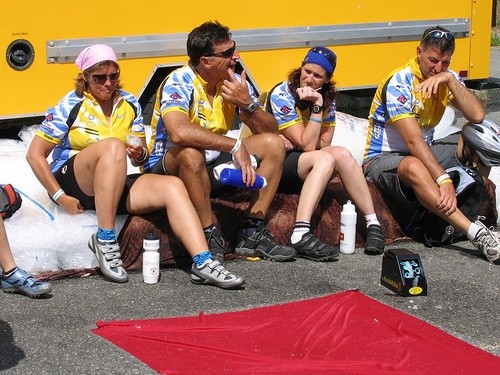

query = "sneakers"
191;259;246;290
287;231;339;261
204;229;227;268
235;226;297;261
1;269;52;298
88;233;129;283
365;224;385;255
471;216;500;261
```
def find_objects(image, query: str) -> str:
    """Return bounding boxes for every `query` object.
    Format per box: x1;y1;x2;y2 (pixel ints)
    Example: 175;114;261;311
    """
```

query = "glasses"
422;29;455;41
85;73;119;84
304;47;336;69
202;40;236;57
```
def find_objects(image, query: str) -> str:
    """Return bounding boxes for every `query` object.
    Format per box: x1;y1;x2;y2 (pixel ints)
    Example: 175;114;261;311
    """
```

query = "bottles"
339;200;357;254
220;168;267;189
213;154;262;181
143;229;160;284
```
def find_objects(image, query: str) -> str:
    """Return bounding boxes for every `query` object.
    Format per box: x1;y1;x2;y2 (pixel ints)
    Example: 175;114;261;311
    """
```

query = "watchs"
244;102;257;113
312;104;323;114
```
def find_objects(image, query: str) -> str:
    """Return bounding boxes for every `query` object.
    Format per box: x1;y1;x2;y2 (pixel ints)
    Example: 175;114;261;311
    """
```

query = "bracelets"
310;116;323;123
52;188;65;202
436;173;453;187
229;138;241;153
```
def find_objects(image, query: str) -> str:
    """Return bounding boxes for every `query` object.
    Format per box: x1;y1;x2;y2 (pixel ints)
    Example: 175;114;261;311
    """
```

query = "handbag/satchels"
0;184;22;221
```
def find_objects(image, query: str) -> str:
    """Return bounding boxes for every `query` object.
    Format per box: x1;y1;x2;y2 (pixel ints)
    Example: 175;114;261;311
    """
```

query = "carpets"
87;289;500;375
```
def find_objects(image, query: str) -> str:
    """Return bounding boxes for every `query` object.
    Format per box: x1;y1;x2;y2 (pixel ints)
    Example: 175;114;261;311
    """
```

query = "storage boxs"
381;248;428;296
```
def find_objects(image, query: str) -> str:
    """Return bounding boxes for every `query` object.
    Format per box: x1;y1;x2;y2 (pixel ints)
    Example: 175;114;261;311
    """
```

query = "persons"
142;21;298;264
27;44;245;289
265;47;384;263
360;27;500;263
0;212;52;299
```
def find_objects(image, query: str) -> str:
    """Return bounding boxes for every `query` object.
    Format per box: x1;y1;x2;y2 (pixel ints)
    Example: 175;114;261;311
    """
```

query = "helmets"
462;119;500;167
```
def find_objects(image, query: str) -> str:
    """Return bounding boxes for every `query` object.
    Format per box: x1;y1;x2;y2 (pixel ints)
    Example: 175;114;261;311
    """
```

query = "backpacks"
405;166;498;247
